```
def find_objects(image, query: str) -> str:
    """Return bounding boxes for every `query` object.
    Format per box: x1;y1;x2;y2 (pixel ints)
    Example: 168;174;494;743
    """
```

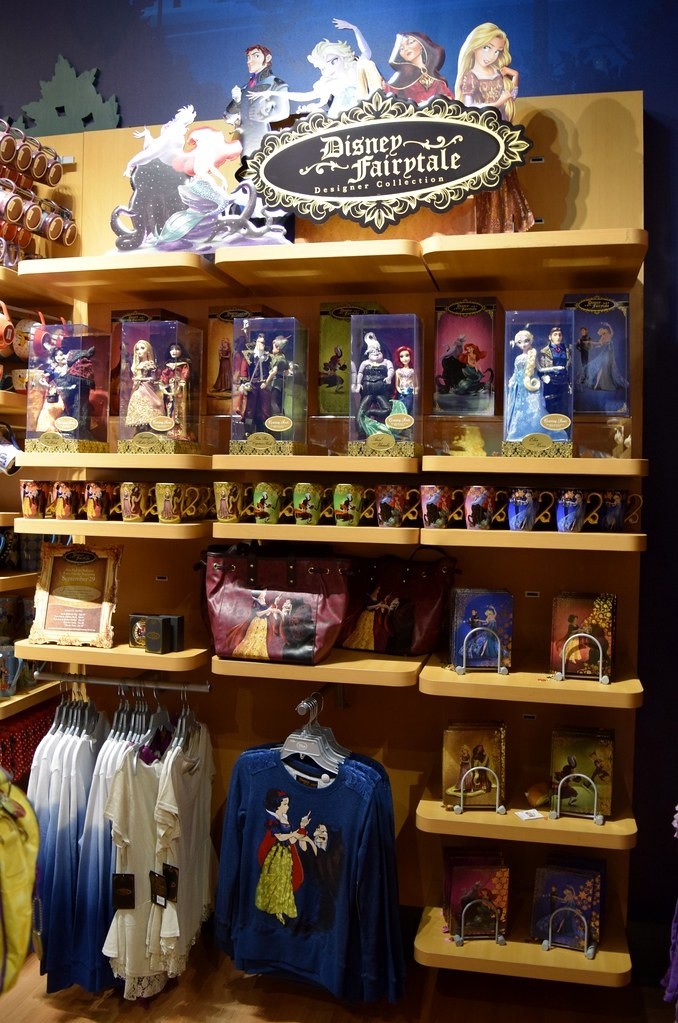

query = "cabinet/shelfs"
1;86;647;1023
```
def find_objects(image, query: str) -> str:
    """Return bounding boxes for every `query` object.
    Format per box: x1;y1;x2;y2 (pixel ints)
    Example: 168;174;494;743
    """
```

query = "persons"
124;322;630;408
225;584;278;660
540;326;573;439
356;332;408;442
235;332;273;441
441;582;612;954
343;583;386;651
260;335;290;440
125;339;165;438
20;480;626;532
36;347;71;433
109;16;537;256
157;339;192;442
55;345;97;441
507;328;569;442
294;810;344;939
272;593;317;665
390;346;420;442
378;595;416;655
256;787;318;926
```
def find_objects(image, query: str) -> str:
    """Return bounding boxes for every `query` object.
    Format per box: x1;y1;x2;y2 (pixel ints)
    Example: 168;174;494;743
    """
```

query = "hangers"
42;673;355;782
